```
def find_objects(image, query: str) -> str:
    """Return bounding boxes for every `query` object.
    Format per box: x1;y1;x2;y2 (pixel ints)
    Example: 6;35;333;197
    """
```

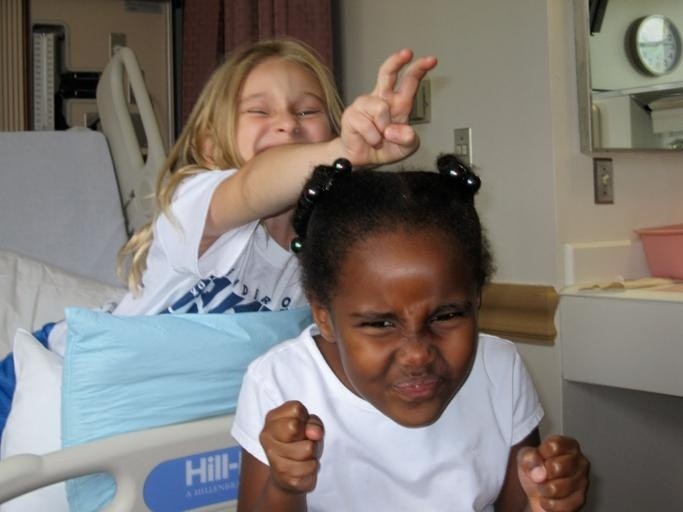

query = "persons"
109;35;440;315
229;154;590;510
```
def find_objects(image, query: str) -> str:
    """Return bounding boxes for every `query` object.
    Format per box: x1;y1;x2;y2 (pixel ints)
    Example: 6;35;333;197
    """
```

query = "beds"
0;47;243;512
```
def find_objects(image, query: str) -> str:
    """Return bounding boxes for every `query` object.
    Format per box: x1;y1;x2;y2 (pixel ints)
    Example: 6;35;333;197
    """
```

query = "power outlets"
594;158;614;204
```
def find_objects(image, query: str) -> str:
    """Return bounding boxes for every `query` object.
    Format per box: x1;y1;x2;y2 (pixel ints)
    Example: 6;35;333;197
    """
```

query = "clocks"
624;14;683;76
